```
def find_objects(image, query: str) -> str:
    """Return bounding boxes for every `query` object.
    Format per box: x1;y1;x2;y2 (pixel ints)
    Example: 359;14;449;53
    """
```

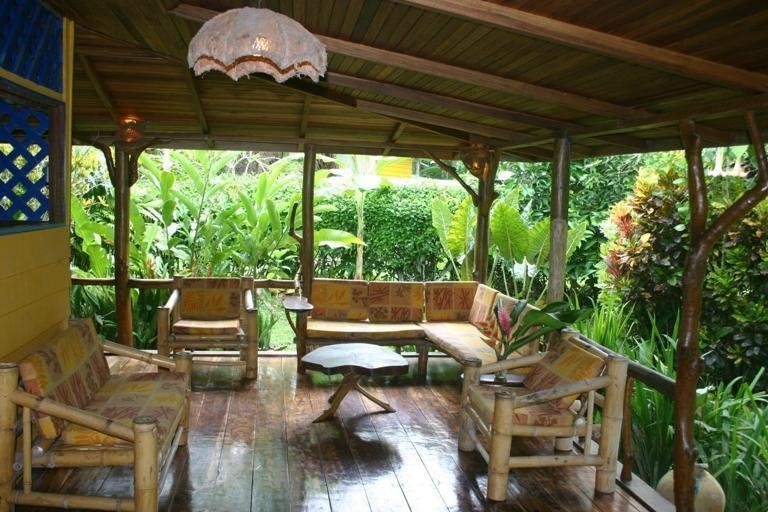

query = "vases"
495;357;508;380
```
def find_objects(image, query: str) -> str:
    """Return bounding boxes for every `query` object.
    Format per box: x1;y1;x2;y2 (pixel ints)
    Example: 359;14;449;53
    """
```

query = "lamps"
186;1;328;83
459;142;490;175
119;117;145;144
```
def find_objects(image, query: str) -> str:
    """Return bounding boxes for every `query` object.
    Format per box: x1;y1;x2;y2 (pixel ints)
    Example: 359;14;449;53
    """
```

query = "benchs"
0;317;191;512
283;278;543;384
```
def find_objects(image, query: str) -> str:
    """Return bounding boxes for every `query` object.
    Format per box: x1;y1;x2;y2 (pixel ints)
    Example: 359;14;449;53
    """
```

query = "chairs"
458;325;628;502
157;276;256;380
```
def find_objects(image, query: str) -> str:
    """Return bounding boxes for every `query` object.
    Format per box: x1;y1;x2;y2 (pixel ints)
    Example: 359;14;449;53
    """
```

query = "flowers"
475;290;592;360
